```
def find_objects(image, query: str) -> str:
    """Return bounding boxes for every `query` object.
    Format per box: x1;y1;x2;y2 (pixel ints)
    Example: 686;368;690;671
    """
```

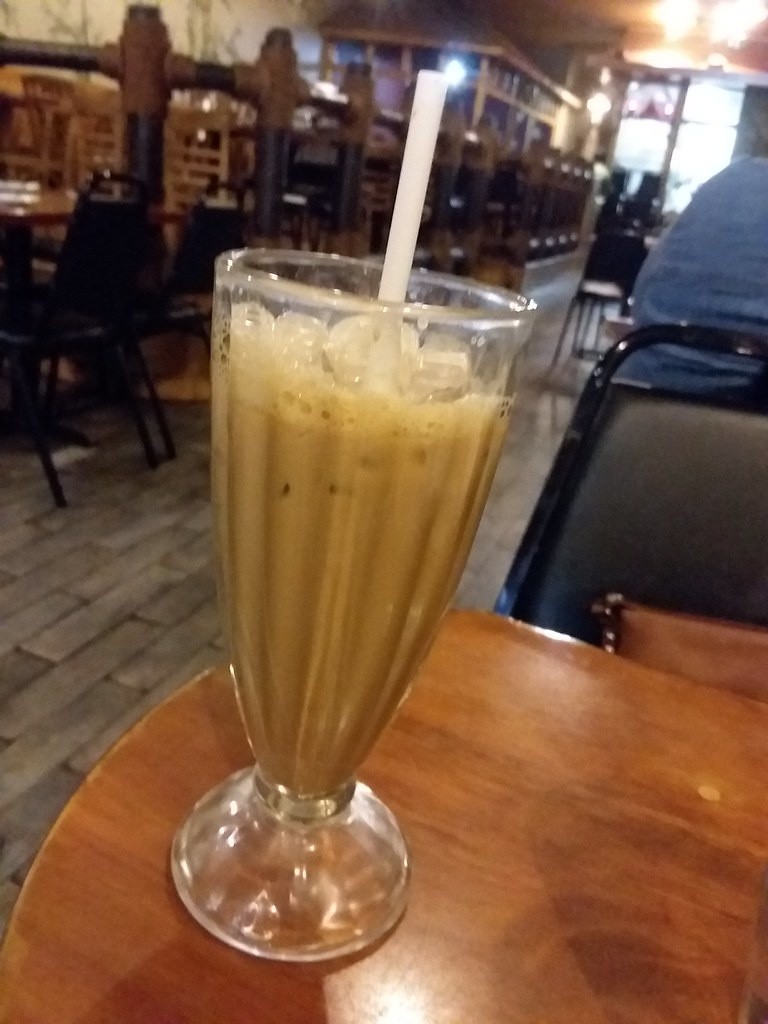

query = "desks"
0;179;78;298
0;605;768;1024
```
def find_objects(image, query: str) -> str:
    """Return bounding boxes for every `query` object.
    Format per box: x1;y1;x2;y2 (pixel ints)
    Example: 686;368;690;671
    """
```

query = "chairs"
0;74;768;694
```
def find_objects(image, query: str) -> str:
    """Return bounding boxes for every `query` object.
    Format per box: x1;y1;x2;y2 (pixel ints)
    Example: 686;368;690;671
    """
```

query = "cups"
165;242;542;963
